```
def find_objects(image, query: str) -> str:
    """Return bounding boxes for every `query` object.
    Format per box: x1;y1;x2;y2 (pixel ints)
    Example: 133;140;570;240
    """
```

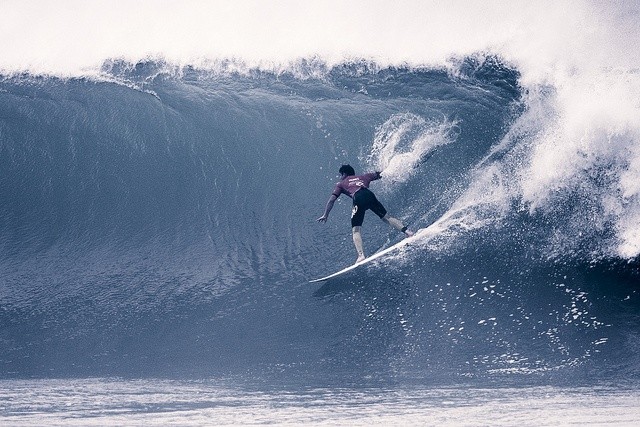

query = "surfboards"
308;212;452;283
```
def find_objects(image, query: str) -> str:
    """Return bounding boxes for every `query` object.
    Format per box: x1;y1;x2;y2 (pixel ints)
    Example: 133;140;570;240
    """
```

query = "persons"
315;165;414;264
